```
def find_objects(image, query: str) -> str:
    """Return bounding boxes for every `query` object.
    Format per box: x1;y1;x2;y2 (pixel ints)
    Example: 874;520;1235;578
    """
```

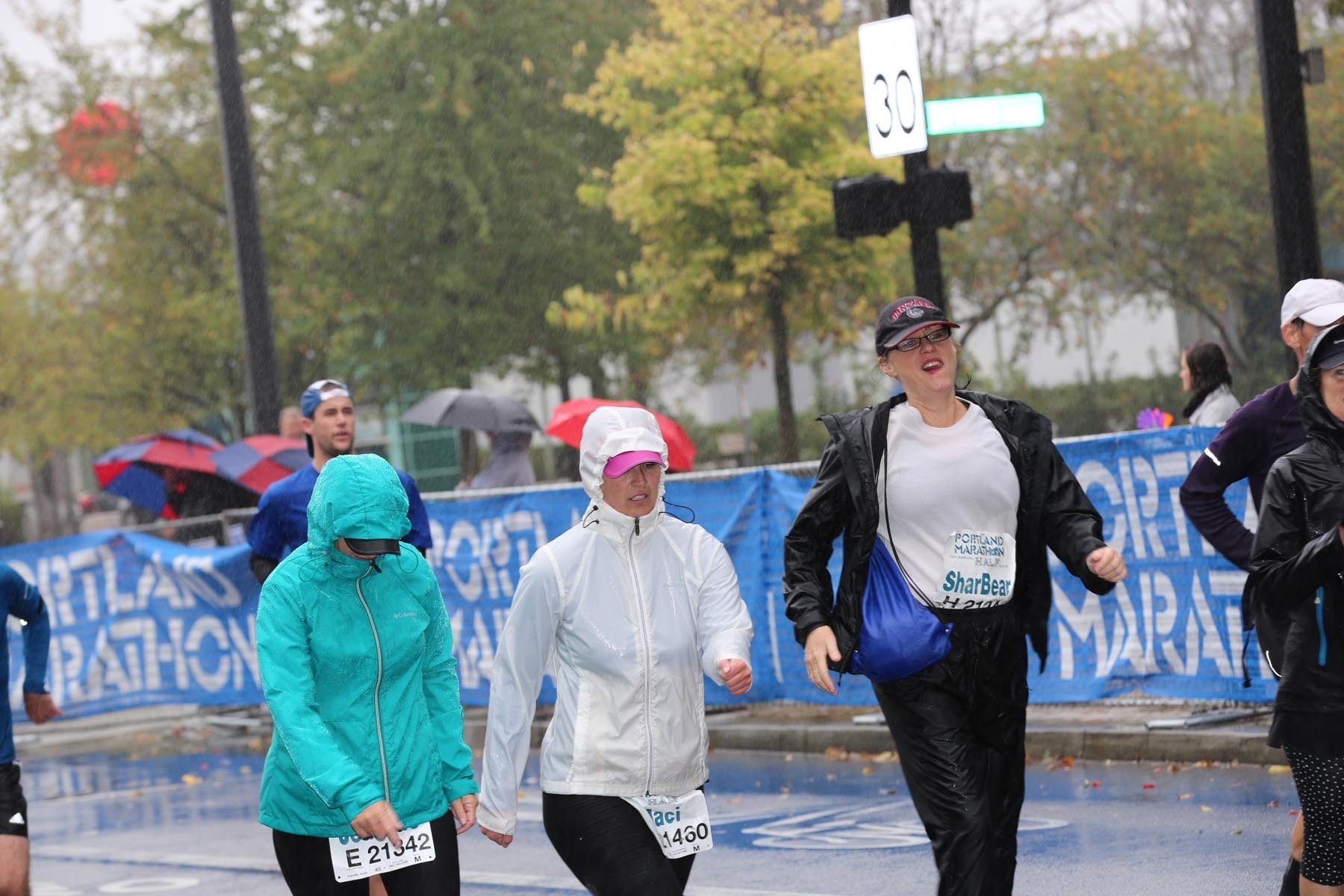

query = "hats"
344;539;402;555
1281;278;1344;328
875;296;961;357
300;379;353;458
603;451;667;479
1311;323;1344;370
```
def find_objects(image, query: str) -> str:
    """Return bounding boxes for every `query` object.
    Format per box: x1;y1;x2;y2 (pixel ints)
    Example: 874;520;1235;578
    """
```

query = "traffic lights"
906;167;973;233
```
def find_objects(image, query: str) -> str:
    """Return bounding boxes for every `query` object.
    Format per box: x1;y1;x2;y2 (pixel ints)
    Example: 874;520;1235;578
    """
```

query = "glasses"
894;327;952;352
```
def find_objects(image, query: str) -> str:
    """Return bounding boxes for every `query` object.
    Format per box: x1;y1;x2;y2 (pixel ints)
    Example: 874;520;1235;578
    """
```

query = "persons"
1180;343;1243;428
0;563;64;896
1179;280;1344;896
155;468;215;518
257;454;478;896
455;430;540;490
1241;324;1344;896
473;407;754;896
781;294;1130;896
245;378;432;586
279;404;318;461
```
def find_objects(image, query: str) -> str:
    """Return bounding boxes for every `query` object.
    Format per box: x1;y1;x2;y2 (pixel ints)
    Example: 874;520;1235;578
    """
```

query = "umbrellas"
546;399;697;475
399;386;545;435
92;425;315;518
